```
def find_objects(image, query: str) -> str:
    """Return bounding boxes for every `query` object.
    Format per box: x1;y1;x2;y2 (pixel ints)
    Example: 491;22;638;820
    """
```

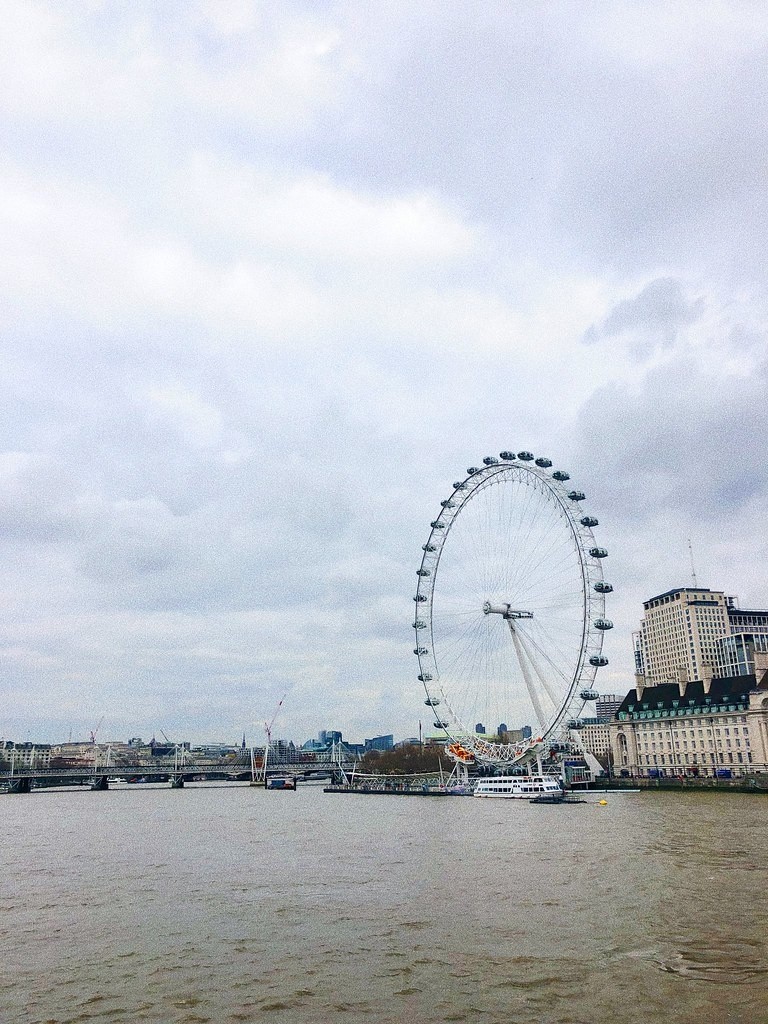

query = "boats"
266;779;295;790
473;776;566;800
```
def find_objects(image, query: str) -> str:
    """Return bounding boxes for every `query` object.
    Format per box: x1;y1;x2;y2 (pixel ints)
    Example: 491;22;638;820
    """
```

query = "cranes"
264;693;287;743
89;716;105;744
